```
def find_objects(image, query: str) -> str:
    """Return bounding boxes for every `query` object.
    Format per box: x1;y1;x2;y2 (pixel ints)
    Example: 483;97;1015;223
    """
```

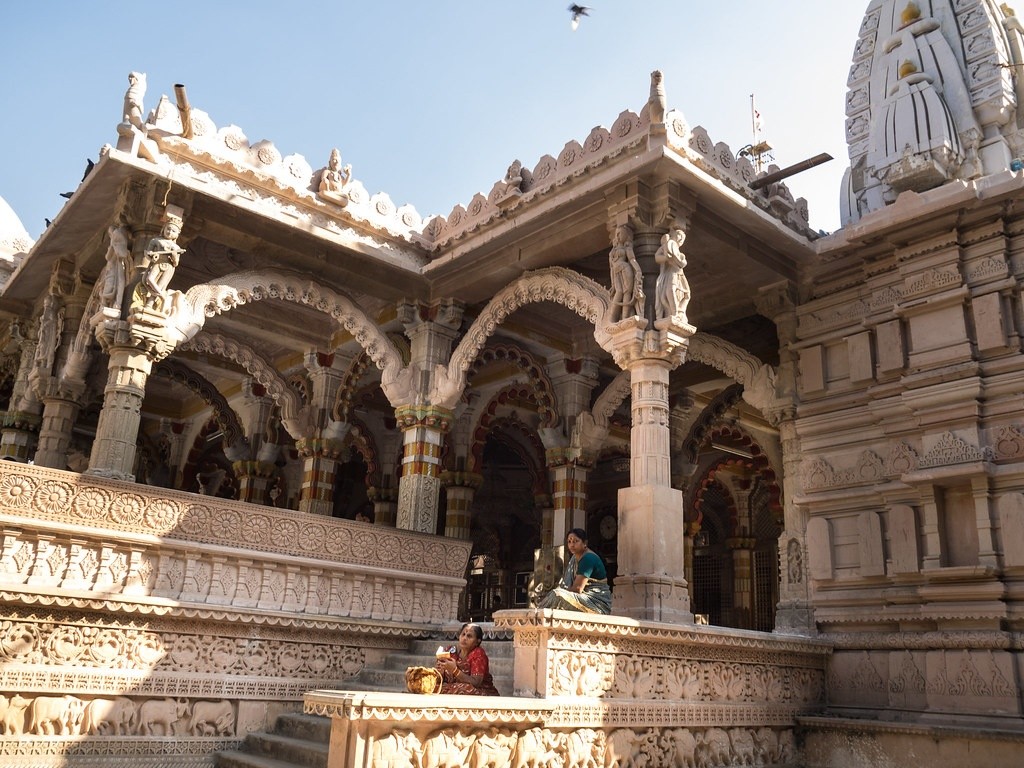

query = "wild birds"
568;3;592;31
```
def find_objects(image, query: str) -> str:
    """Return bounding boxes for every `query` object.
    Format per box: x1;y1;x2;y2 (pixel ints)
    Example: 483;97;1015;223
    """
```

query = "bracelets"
452;667;462;679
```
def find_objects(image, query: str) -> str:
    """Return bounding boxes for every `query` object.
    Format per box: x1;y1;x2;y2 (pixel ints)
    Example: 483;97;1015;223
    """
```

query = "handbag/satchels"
405;666;443;695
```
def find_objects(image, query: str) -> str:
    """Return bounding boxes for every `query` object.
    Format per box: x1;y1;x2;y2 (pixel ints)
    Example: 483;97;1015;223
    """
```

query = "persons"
31;218;188;378
433;623;499;696
317;149;356;206
602;223;692;326
534;527;612;615
